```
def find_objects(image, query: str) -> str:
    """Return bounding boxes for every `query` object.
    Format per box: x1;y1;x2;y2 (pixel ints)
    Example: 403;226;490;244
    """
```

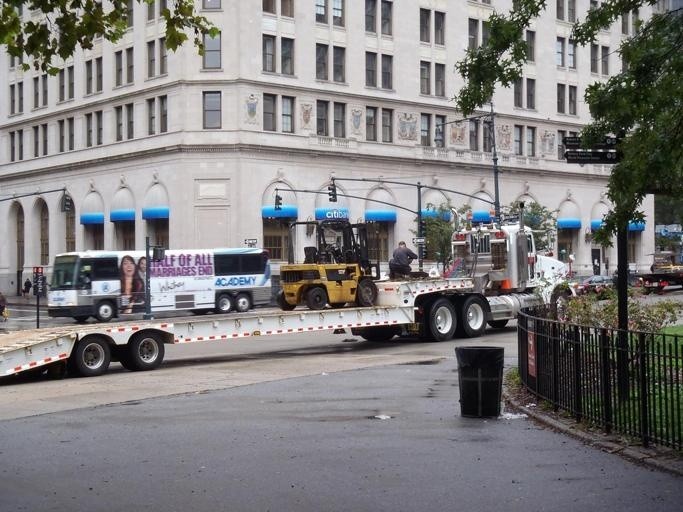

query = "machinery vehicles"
275;216;382;312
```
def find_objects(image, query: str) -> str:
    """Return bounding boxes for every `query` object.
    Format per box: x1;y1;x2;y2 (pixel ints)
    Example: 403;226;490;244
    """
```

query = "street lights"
433;112;501;223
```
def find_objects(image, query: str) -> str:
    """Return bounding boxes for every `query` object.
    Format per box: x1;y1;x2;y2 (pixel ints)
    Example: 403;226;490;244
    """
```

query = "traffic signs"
559;136;617;150
562;151;618;164
411;237;426;244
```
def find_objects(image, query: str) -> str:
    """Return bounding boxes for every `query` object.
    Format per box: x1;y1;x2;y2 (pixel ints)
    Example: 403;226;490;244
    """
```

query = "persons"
387;241;417;283
23;278;32;300
137;256;149;311
0;291;8;323
118;254;145;316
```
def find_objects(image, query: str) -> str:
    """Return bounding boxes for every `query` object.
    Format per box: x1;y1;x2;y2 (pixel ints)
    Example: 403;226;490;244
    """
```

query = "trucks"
0;219;573;384
635;272;682;295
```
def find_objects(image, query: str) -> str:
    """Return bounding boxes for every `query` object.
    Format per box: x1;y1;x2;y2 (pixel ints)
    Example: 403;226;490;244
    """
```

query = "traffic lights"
274;194;283;211
422;245;429;259
420;220;427;236
328;184;337;203
62;195;71;213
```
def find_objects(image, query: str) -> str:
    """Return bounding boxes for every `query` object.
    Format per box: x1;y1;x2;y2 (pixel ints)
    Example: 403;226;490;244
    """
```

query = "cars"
574;274;617;300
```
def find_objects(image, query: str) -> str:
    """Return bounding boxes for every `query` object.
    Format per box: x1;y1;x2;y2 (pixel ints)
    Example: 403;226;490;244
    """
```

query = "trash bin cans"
455;346;504;418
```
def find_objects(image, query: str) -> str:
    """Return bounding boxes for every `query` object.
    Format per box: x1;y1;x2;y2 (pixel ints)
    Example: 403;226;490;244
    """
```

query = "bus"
46;246;274;324
